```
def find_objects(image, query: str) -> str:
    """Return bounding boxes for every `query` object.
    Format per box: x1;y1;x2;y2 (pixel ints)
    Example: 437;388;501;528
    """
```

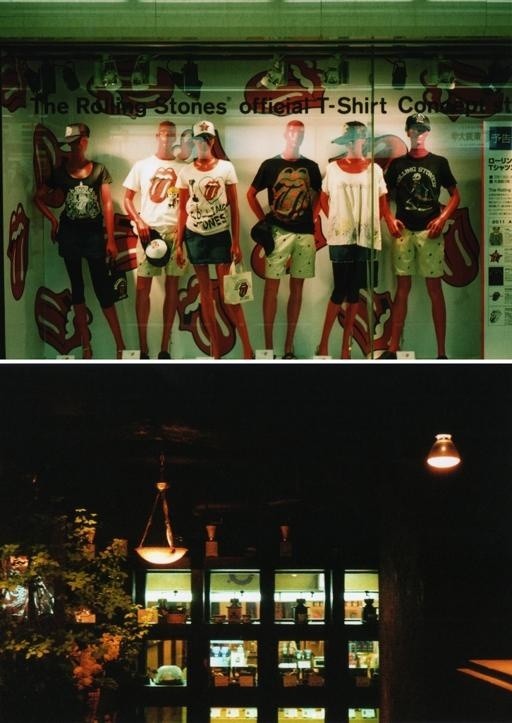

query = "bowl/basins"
213;614;225;624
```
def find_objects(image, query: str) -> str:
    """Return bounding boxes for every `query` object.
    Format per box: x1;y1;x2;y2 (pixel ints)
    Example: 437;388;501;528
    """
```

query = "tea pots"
158;605;186;624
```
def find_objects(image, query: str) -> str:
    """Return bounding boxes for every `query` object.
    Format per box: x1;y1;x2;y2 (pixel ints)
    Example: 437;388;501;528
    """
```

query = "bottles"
225;599;240;622
361;599;376;624
157;599;167;622
282;643;287;654
289;644;293;654
293;599;309;624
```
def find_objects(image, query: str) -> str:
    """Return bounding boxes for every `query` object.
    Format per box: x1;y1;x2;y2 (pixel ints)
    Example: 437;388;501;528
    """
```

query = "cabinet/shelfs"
136;569;382;723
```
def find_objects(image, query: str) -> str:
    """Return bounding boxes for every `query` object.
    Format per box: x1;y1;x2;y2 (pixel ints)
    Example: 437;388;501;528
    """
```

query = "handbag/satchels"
223;271;254;305
108;270;128;302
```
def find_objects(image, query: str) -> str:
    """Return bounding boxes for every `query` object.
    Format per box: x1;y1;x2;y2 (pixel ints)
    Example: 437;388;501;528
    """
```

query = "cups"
304;650;312;659
242;614;251;624
295;651;302;660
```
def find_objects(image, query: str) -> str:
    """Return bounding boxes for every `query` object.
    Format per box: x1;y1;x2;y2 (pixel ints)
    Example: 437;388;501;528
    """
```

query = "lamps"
427;397;463;471
133;445;190;567
206;525;218;557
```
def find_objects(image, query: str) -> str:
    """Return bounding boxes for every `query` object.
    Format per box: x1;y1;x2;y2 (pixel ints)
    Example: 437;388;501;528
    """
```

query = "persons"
1;542;55;619
121;120;191;361
317;120;391;361
173;119;259;361
247;116;324;360
378;111;462;360
32;122;128;361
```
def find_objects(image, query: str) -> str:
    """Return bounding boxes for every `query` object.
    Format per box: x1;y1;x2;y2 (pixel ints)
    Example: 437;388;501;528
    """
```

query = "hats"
406;113;431;131
141;230;170;267
251;219;274;256
331;122;367;145
193;120;216;138
57;124;89;144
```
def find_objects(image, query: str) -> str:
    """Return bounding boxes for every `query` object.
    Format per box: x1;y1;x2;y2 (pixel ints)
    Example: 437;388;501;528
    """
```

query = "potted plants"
0;503;162;723
